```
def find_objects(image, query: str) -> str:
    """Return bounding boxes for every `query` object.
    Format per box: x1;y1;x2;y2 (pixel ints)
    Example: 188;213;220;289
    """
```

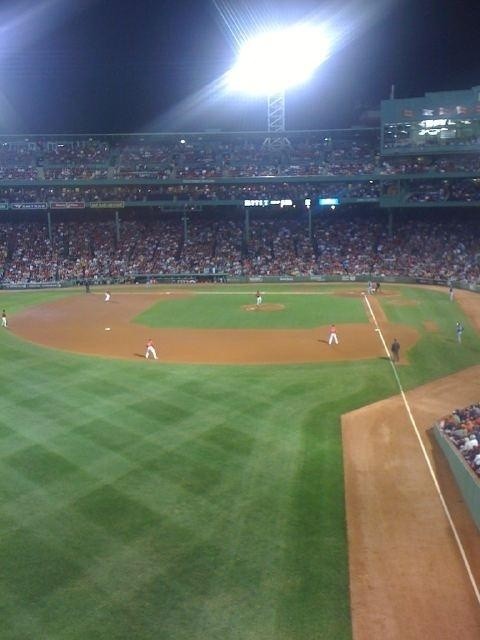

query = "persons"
449;285;455;302
256;289;263;305
2;309;7;328
439;403;480;478
328;324;338;345
145;337;158;360
105;290;113;303
1;136;480;292
368;278;381;294
457;320;464;343
391;337;401;361
